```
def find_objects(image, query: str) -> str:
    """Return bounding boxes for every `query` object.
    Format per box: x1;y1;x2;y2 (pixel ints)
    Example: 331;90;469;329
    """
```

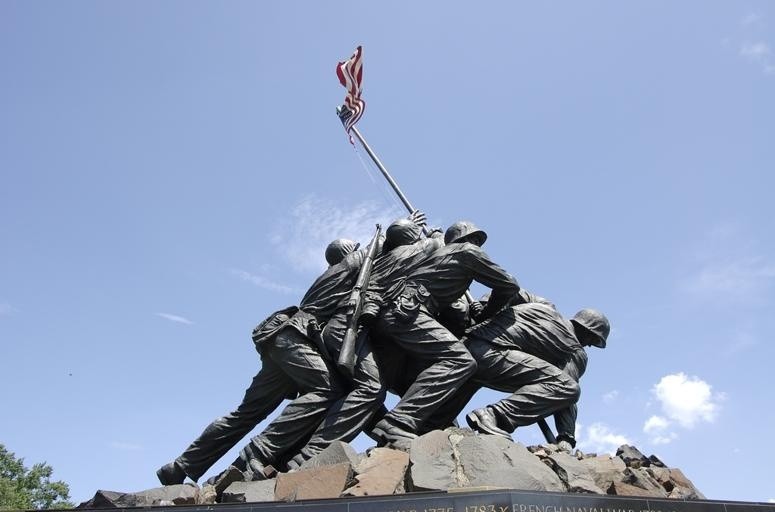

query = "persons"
414;285;611;449
155;208;520;502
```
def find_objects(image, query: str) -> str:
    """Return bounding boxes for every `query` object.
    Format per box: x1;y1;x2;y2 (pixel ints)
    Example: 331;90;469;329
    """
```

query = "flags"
334;45;366;146
